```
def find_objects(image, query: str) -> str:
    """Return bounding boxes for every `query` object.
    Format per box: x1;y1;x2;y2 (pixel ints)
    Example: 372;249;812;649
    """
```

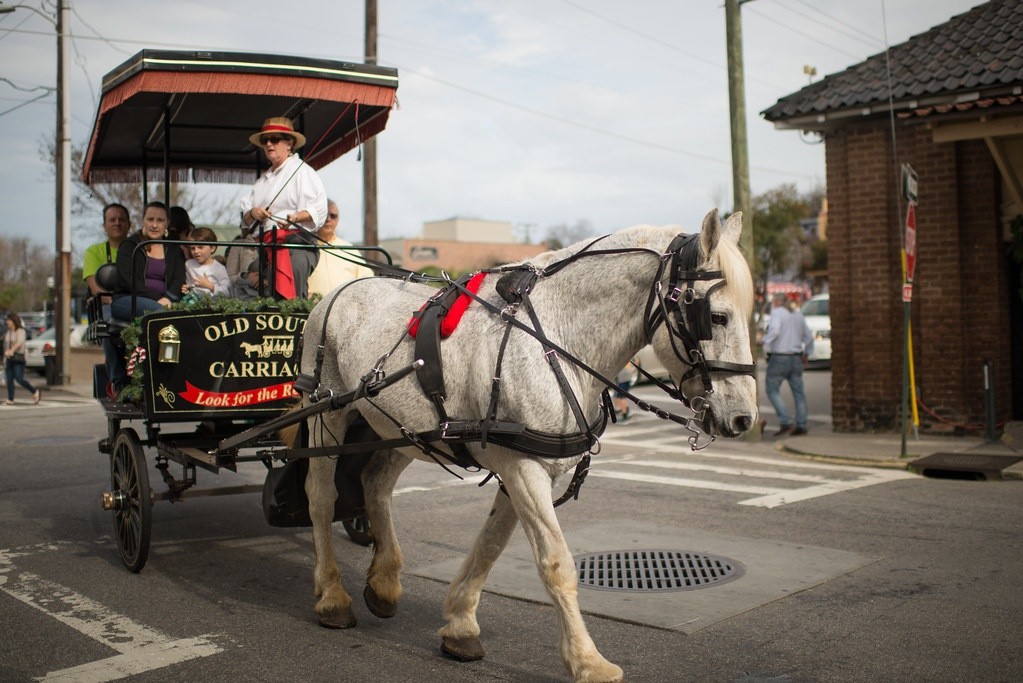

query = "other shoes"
106;379;119;402
790;426;807;436
2;401;14;405
773;424;796;436
34;389;41;404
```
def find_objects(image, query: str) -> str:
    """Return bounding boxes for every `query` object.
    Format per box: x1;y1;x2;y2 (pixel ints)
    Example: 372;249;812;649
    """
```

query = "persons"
613;367;632;421
224;220;269;290
307;199;375;301
170;206;194;259
110;200;188;322
181;227;231;299
240;117;328;300
760;293;814;435
0;312;41;405
82;202;131;319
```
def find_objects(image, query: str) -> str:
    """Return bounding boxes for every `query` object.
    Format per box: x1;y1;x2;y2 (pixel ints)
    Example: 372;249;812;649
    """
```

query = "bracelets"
294;215;297;223
250;208;256;220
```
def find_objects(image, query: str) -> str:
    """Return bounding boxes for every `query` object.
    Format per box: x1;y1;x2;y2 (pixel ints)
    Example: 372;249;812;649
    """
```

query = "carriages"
77;48;761;683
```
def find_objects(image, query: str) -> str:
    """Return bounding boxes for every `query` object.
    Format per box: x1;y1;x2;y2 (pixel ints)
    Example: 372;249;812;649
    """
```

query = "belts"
772;352;802;356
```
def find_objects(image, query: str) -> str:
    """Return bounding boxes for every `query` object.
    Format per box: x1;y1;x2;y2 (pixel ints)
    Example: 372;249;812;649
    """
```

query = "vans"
754;279;811;345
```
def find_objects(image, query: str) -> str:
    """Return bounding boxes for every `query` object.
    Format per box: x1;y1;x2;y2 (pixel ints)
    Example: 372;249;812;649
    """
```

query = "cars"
22;323;88;377
796;292;833;370
1;311;56;356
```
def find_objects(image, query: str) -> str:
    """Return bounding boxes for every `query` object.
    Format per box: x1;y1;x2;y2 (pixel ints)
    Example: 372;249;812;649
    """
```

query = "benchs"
85;291;137;344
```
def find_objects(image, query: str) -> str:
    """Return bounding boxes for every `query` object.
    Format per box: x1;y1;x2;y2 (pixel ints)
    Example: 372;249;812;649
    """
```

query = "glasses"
330;214;338;220
260;136;289;145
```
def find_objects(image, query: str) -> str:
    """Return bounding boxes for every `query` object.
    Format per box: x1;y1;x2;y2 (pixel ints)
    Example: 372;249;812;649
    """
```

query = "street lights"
45;274;56;328
0;1;73;390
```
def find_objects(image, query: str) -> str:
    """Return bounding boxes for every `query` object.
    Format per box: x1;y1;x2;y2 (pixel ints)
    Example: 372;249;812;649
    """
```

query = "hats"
248;116;306;149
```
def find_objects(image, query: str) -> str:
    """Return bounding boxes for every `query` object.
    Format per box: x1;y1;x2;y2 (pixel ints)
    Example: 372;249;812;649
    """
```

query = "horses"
309;209;759;683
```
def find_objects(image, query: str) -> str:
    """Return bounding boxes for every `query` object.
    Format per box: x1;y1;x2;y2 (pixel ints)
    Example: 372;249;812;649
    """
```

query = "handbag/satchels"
13;352;25;363
